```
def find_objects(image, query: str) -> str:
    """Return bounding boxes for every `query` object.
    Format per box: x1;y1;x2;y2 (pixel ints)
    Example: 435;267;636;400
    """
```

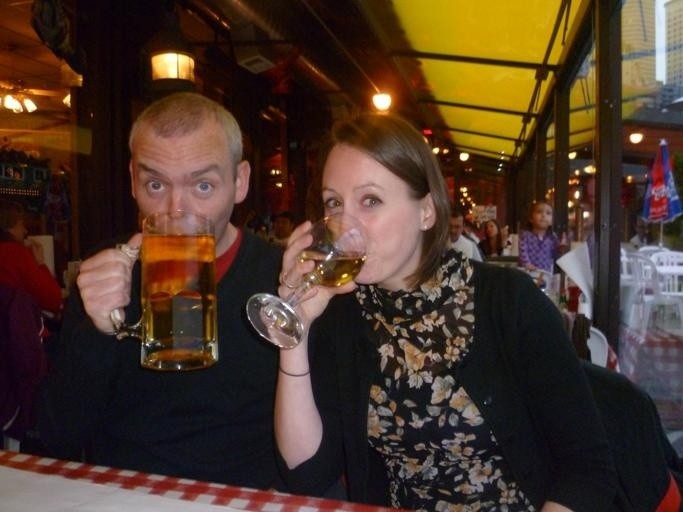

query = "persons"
514;199;565;288
0;192;68;451
232;206;256;228
0;89;341;498
475;218;504;261
627;208;657;251
441;205;484;265
265;210;296;249
258;108;623;509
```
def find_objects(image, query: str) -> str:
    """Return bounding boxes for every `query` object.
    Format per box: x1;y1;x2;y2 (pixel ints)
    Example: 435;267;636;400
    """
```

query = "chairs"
518;266;562;304
563;318;609;370
618;241;683;337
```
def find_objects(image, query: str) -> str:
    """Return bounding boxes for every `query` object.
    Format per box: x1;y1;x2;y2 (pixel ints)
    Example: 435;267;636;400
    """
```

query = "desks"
0;449;417;512
481;252;519;269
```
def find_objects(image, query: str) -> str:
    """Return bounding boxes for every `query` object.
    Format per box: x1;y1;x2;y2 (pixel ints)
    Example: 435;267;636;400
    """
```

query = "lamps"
372;93;392;111
138;48;196;104
0;42;39;114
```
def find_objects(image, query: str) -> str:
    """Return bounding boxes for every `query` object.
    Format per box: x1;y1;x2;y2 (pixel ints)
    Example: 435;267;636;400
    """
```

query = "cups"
109;211;218;371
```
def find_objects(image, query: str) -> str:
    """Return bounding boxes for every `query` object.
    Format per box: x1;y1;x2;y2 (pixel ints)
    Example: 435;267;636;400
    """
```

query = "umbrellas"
639;138;682;250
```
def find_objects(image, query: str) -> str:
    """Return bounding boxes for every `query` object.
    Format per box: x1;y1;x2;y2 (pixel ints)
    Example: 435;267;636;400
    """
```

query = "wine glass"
246;213;368;348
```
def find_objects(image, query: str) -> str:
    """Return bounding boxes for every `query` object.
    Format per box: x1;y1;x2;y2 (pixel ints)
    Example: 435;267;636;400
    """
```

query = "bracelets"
278;367;311;378
